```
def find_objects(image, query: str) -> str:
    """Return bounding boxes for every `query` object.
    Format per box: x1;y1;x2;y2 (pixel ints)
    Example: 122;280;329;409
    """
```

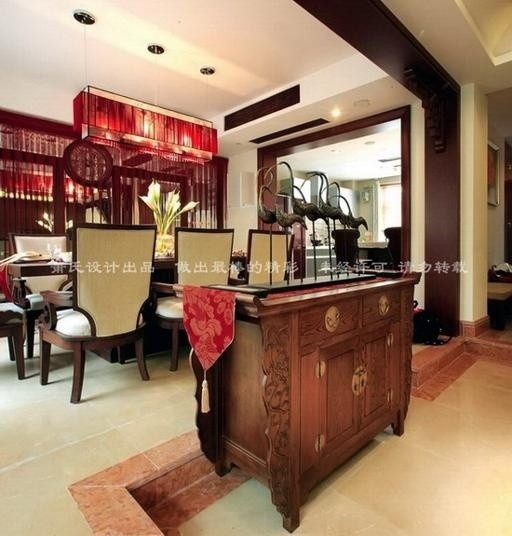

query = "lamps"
71;11;220;168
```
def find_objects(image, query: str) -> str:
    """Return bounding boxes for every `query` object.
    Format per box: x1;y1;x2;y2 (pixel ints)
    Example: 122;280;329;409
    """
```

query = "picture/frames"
488;138;501;209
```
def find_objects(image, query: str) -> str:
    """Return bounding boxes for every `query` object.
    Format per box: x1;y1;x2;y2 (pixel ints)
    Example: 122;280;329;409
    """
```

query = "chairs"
0;214;297;407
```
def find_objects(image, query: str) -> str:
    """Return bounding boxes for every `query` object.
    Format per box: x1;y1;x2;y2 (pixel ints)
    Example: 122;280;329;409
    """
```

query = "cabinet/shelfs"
188;268;422;532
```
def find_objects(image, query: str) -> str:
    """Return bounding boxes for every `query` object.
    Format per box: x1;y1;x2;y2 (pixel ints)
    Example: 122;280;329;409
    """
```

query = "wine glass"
47;244;57;264
